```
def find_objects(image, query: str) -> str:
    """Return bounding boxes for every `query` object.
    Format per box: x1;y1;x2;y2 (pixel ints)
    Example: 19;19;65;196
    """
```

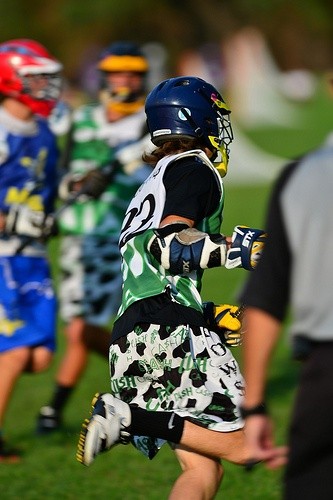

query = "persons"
239;129;333;499
75;75;266;500
0;40;159;461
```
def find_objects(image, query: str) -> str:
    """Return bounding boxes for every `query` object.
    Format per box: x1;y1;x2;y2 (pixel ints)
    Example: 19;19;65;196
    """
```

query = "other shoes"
38;404;60;430
0;437;24;464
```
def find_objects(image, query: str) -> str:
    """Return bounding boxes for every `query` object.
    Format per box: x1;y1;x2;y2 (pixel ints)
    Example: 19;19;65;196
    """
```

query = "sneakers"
76;392;132;465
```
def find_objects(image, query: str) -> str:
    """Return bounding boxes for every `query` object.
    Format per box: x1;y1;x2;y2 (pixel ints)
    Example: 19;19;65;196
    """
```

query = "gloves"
224;223;272;272
115;134;159;175
4;201;61;241
62;173;104;200
203;301;246;348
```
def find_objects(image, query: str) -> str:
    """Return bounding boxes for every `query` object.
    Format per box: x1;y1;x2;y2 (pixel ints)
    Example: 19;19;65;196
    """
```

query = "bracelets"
239;401;267;419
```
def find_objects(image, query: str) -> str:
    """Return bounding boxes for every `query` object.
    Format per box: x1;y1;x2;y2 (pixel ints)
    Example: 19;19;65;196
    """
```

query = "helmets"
95;43;150;123
1;38;64;118
146;71;235;180
